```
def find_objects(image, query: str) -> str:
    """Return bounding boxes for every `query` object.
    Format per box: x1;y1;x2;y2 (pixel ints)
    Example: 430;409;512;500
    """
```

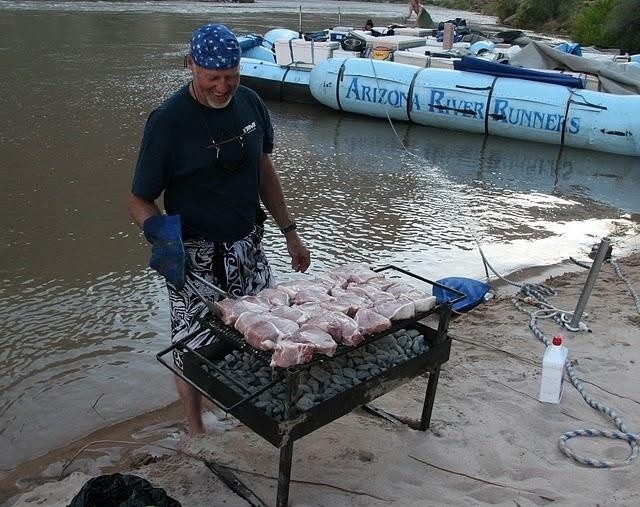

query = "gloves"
141;213;195;291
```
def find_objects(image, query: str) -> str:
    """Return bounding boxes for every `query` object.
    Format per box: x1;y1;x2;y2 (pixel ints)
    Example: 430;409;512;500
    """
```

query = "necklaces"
190;78;235;161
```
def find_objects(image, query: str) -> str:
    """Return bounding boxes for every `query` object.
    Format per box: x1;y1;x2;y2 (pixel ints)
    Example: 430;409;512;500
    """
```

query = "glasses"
209;125;250;172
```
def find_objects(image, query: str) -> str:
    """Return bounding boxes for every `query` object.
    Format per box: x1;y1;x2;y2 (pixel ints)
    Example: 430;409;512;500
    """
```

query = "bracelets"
280;220;297;234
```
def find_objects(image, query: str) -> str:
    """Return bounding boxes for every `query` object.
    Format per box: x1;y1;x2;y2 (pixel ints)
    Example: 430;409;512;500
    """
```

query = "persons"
127;23;311;436
404;0;435;28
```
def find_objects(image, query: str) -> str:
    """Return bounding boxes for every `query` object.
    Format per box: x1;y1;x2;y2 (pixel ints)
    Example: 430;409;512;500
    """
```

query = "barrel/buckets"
371;45;393;60
344;32;361;50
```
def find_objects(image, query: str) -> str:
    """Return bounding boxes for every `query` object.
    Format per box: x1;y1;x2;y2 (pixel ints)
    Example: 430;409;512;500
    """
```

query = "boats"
236;17;640;156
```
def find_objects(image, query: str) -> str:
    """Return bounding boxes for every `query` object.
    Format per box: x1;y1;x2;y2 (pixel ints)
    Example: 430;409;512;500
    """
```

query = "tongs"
182;271;238;317
